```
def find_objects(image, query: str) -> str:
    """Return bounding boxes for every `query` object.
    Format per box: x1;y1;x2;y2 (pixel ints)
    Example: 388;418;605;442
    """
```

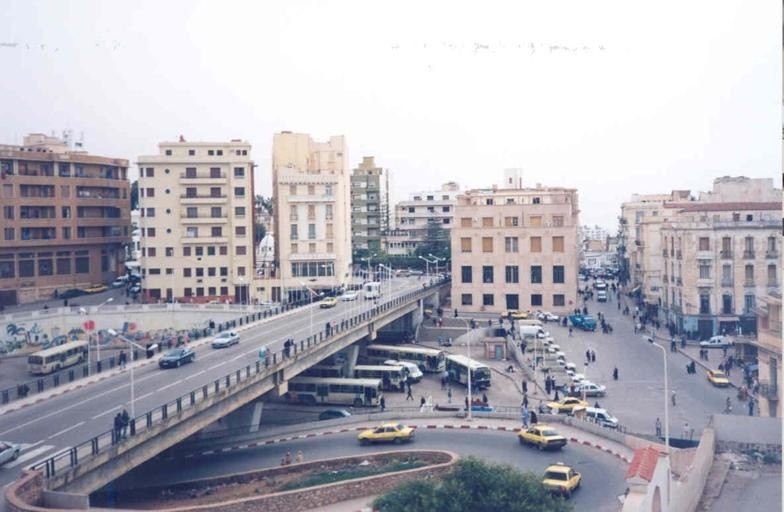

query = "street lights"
300;282;326;337
640;334;673;493
419;256;437;284
452;315;472;420
377;262;392;300
78;298;116;372
428;252;447;277
360;252;378;281
107;325;162;426
235;264;286;319
320;262;333;294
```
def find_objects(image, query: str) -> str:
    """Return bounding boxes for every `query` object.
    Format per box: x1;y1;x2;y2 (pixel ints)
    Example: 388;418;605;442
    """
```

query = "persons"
381;385;425;411
575;282;593;314
487;310;526;354
611;282;633;315
326;322;330;337
613;368;618;380
520;374;581;428
543;315;573;336
663;322;709;374
598;312;613;333
120;350;127;369
441;376;452;402
719;328;760;415
432;305;479;329
586;349;595;362
464;385;487;405
114;409;130;443
632;302;659;334
284;339;294;358
655;393;689;438
281;449;303;466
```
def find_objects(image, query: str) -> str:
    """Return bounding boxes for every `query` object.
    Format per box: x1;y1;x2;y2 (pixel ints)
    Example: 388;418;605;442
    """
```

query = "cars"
212;330;241;348
705;370;730;388
434;272;451;282
316;409;351;424
500;308;617;430
319;267;423;310
359;421;417;444
578;266;619;303
0;439;22;471
157;347;195;369
700;335;728;349
425;307;435;316
58;273;139;299
514;423;567;449
201;300;224;309
539;460;582;498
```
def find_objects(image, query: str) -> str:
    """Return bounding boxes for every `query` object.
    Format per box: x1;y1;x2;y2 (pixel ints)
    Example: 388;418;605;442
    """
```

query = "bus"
268;344;493;407
27;339;89;375
